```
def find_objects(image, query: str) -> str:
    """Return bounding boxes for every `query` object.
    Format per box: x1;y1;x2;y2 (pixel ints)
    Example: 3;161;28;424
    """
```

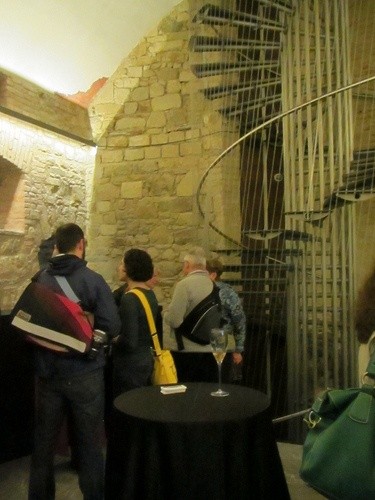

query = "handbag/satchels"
299;352;375;500
150;350;178;386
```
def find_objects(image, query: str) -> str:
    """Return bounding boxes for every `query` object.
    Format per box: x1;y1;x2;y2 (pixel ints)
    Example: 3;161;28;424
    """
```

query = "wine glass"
210;329;230;397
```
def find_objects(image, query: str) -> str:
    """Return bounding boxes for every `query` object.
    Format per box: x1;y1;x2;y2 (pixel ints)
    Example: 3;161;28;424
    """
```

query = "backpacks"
174;281;224;346
9;268;93;354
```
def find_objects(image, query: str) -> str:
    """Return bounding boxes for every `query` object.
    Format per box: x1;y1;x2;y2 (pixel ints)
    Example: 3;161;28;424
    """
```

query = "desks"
105;383;291;500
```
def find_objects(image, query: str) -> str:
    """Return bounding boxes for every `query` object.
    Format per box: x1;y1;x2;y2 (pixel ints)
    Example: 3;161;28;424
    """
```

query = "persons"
28;222;122;500
106;247;161;444
162;246;220;384
205;259;247;386
